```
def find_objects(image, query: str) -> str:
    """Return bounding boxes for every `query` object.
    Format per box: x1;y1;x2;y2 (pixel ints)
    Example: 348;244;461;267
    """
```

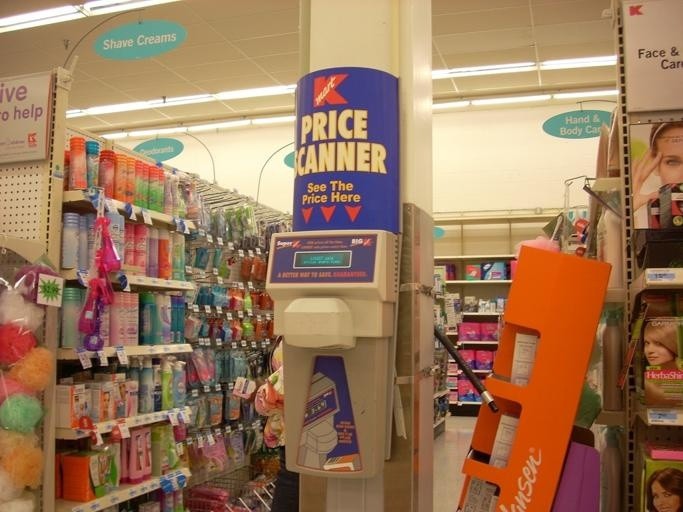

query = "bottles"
58;213;185;283
60;358;185;428
61;282;186;350
65;425;183;510
65;138;185;220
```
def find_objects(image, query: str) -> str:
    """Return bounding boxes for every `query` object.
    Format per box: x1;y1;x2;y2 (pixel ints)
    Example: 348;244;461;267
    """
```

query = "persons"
631;122;681;230
641;320;679;403
644;467;683;512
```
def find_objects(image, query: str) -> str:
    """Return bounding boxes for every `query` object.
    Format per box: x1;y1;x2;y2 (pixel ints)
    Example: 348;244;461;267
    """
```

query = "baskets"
184;463;280;511
